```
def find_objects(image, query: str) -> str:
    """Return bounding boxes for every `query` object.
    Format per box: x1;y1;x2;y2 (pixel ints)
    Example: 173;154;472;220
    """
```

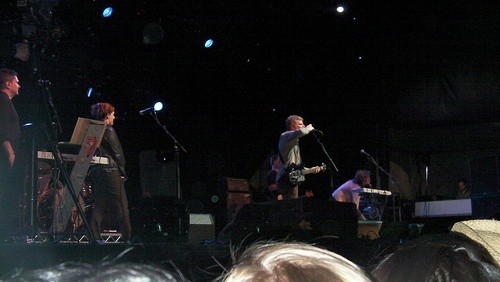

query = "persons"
216;238;373;282
303;186;318;200
88;102;132;242
267;152;280;199
368;231;500;281
278;113;322;199
0;69;23;241
327;170;371;221
456;178;477;216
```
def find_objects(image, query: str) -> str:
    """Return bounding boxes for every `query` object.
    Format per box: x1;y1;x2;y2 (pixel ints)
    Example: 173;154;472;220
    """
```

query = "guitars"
285;163;326;185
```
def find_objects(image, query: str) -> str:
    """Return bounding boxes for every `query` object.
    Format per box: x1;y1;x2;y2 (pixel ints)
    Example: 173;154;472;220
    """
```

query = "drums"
36;186;91;233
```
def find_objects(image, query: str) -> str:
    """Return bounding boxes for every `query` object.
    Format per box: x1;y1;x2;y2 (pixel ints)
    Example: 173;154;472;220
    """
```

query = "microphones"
37;79;51;87
139;107;154;113
361;150;371;157
313;128;322;133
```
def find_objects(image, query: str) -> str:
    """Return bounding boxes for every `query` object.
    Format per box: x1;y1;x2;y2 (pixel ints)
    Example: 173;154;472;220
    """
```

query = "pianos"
37;151;109;164
356;187;392;195
